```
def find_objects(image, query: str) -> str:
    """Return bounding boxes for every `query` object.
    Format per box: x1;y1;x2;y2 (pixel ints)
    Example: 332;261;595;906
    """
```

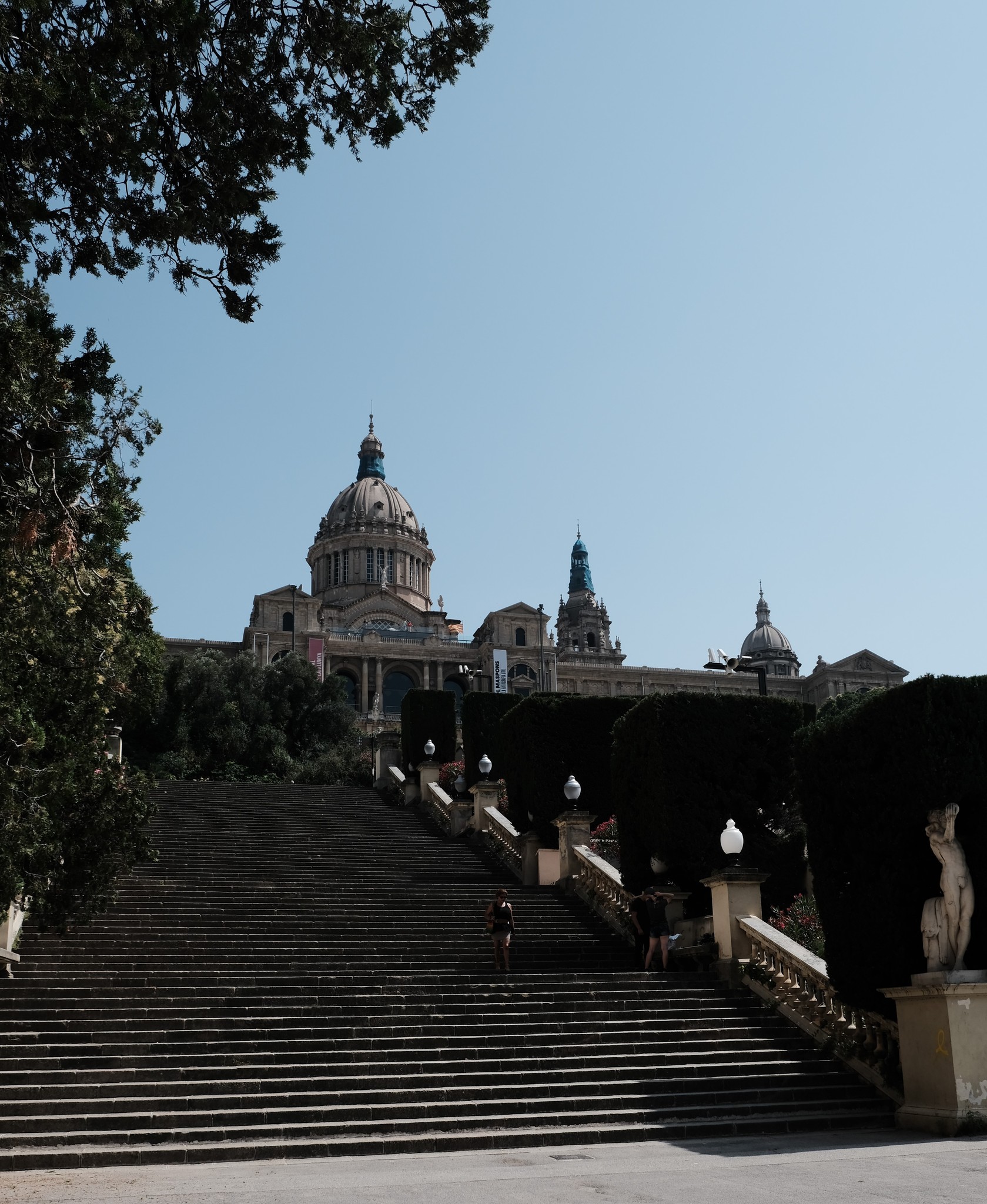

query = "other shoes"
644;968;649;972
662;968;667;972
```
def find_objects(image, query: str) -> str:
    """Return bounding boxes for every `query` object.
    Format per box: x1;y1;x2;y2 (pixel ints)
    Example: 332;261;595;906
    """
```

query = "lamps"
478;754;492;782
563;775;582;811
424;739;436;761
720;818;744;866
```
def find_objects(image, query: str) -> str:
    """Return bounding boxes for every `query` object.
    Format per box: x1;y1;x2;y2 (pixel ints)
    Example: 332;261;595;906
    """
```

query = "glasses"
499;896;507;899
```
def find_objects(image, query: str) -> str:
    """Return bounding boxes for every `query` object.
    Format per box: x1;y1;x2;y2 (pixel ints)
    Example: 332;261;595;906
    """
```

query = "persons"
630;888;656;972
925;802;975;970
484;889;515;974
644;891;674;972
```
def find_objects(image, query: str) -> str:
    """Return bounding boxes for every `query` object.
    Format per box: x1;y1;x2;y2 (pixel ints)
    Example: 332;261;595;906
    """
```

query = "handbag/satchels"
668;934;683;950
486;921;493;934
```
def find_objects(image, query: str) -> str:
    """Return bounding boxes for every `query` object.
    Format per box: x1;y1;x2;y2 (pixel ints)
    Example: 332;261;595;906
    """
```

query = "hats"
644;888;655;895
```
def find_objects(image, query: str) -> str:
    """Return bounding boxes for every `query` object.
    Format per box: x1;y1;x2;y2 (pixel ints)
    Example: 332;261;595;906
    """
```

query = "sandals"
505;964;511;971
495;963;500;970
